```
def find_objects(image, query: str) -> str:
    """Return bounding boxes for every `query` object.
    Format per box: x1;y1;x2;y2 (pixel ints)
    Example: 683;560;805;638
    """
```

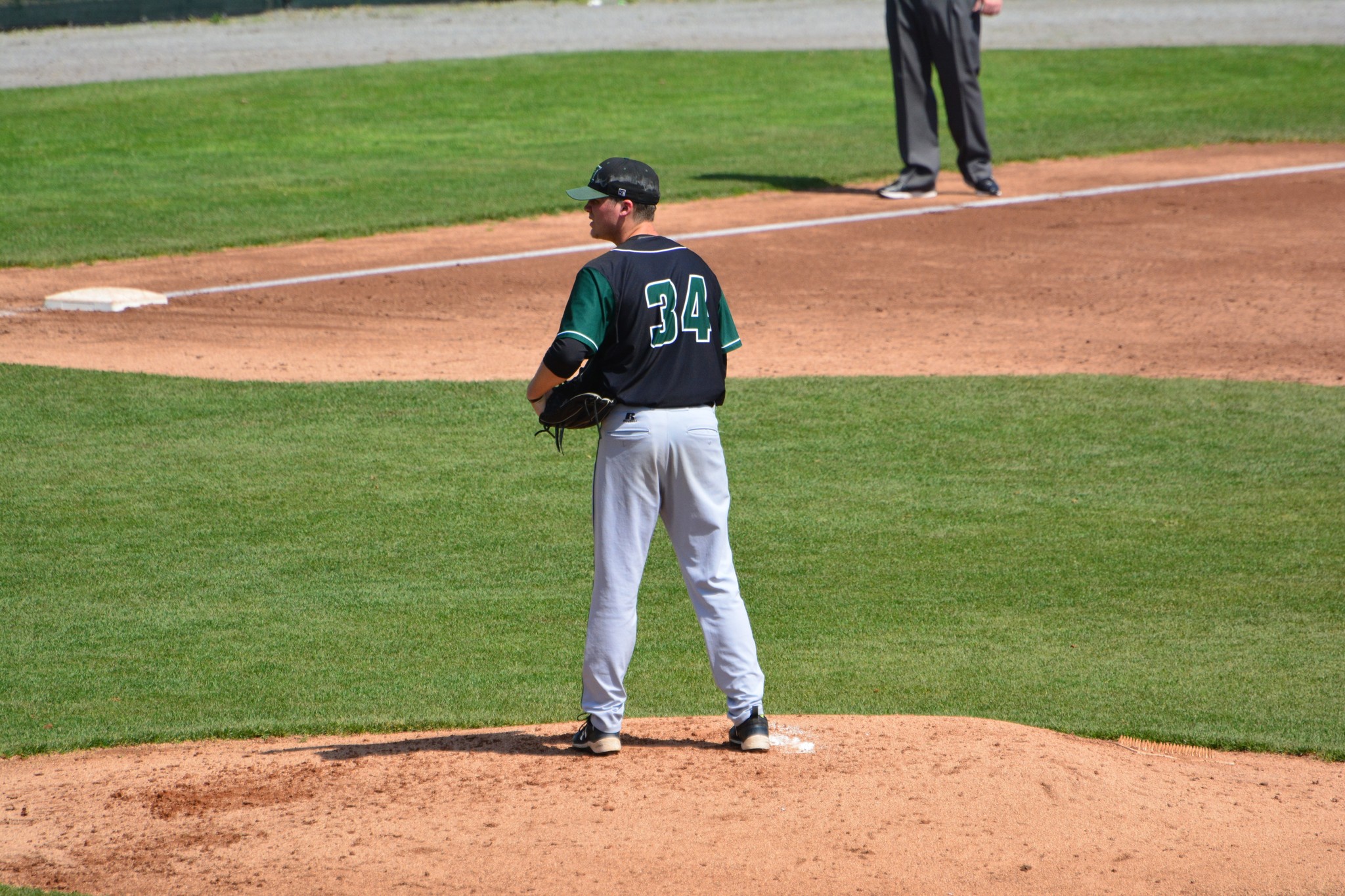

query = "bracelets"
527;394;544;403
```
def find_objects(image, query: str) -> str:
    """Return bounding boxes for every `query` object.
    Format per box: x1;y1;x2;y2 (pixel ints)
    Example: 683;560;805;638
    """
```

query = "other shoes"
975;177;998;196
880;180;937;200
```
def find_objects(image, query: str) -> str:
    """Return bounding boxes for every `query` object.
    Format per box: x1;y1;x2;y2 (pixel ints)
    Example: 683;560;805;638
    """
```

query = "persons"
876;0;1005;199
527;158;769;754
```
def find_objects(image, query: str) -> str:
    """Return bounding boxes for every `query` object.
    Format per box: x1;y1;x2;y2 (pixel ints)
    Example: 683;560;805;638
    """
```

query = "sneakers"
730;705;771;752
568;712;622;754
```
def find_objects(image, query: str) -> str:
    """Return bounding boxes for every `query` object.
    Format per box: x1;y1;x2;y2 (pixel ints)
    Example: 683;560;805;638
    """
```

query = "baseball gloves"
538;380;615;430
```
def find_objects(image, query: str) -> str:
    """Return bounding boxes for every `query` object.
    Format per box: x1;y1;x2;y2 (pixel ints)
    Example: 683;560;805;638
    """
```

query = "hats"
566;156;660;205
